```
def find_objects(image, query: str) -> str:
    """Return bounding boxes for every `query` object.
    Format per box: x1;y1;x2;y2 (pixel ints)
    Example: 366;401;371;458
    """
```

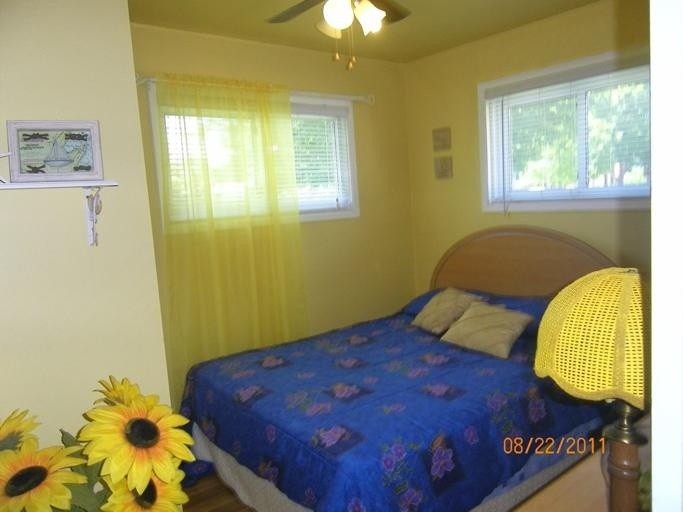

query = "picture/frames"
5;120;105;183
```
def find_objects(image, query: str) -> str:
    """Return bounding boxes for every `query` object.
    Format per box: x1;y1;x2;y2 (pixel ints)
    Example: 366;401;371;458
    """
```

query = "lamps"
535;267;651;511
314;1;387;73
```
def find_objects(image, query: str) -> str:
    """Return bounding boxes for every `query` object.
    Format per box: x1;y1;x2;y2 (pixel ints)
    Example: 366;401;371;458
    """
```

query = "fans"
264;1;412;25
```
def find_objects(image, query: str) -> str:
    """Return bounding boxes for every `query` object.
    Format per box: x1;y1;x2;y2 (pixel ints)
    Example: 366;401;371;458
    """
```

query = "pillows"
401;283;551;360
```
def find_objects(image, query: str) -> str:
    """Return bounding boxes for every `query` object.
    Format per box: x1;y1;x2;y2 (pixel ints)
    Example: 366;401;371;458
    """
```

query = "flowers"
0;375;195;511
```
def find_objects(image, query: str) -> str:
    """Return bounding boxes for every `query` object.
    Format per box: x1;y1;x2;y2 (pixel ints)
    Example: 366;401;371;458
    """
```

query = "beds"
180;226;618;511
